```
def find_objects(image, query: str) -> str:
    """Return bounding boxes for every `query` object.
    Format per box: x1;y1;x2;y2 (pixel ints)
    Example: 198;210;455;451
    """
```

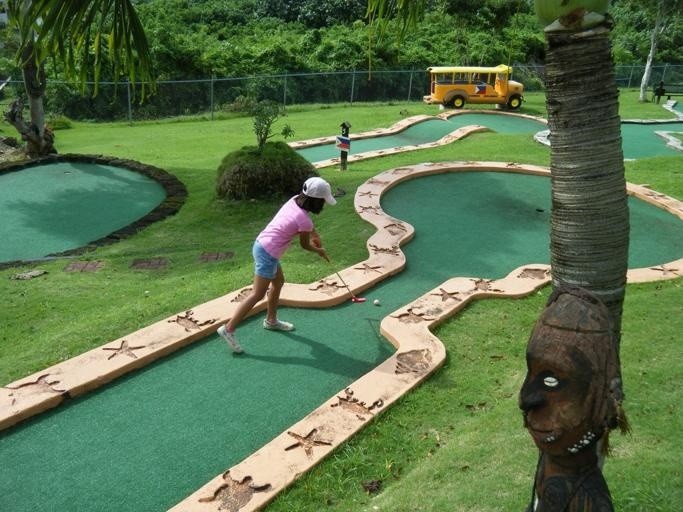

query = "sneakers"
263;318;295;331
216;323;244;354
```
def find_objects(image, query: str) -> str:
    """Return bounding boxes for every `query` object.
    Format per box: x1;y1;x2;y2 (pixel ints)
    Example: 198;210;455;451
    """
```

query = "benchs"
651;83;683;103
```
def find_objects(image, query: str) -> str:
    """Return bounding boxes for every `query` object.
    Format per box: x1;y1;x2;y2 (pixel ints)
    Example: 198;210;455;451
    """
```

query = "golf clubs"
310;237;366;303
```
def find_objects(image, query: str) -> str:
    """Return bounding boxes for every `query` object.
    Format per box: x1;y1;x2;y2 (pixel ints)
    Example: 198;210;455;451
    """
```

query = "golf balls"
373;299;379;305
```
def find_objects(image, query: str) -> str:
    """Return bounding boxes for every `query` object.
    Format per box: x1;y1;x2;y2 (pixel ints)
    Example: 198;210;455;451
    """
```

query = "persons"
215;175;338;356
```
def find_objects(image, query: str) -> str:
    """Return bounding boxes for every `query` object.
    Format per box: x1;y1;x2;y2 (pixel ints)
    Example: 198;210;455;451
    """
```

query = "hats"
302;177;337;206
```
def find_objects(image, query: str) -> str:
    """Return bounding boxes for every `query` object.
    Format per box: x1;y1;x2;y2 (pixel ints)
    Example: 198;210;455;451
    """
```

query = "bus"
422;64;524;109
